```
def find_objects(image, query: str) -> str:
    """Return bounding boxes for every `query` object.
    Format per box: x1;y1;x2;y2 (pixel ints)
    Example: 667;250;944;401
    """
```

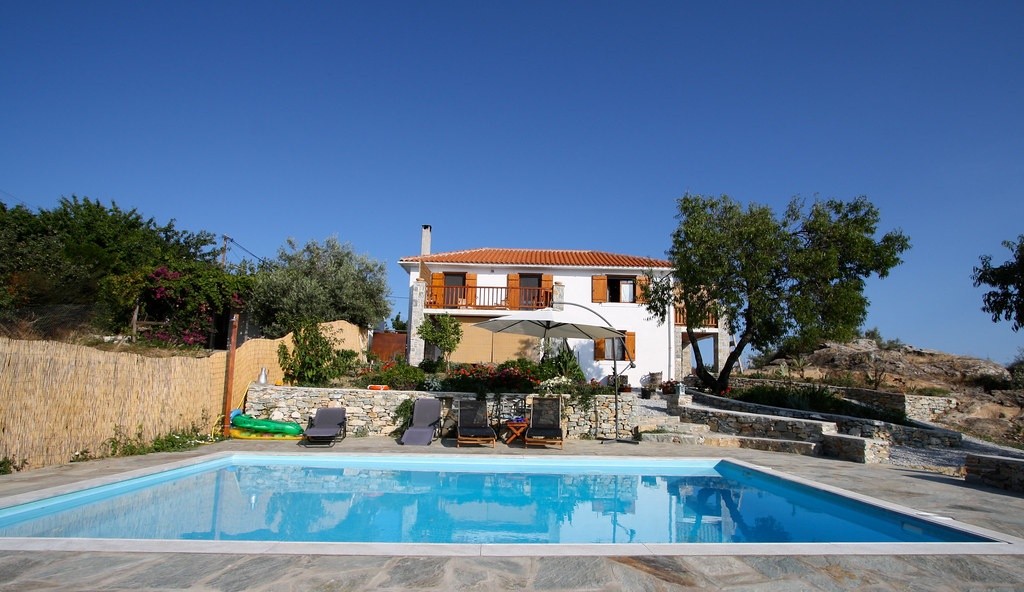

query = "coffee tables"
505;422;528;444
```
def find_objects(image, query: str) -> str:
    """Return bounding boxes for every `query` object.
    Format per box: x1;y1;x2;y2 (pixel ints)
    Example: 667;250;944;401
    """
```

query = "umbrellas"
469;300;641;447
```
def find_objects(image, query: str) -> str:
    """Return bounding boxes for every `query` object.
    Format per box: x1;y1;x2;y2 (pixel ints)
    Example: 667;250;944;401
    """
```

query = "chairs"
302;407;347;444
457;400;497;448
401;398;442;446
525;397;563;449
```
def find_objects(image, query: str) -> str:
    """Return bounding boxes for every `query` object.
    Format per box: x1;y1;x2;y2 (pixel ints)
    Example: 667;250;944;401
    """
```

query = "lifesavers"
367;384;388;389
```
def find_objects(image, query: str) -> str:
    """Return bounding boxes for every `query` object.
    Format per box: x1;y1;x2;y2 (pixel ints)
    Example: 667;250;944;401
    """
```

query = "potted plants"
618;383;631;392
659;378;679;395
641;373;650;399
